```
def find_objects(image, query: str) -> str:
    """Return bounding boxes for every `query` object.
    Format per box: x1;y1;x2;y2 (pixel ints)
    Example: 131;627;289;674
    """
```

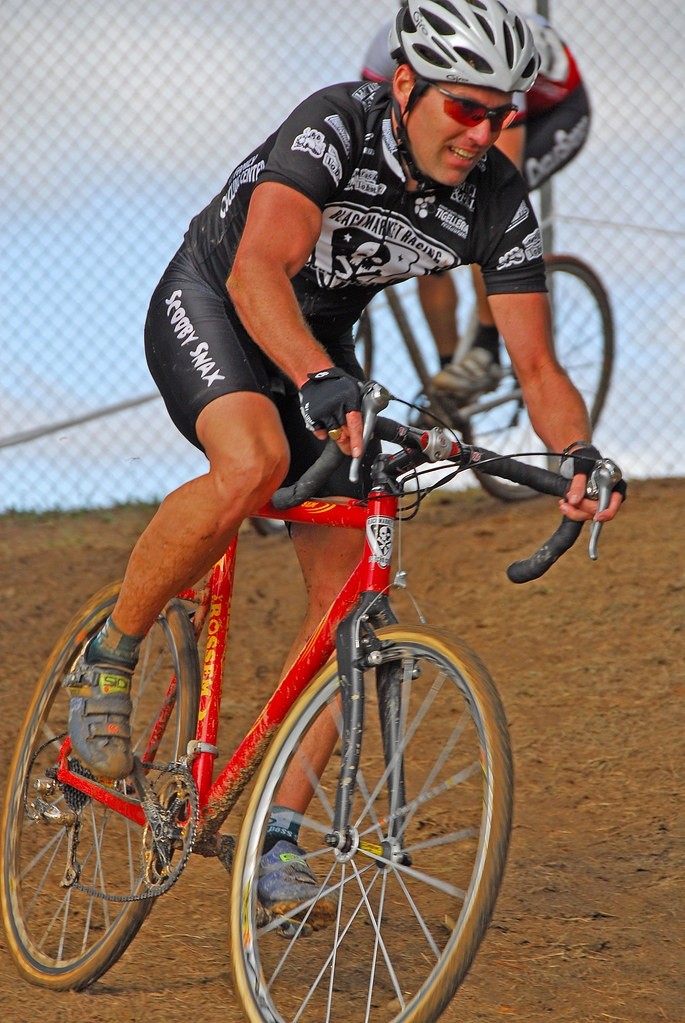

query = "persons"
359;8;592;410
58;0;628;931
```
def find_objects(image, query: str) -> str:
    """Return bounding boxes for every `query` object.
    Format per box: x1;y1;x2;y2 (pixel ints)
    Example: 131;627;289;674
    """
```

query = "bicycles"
343;253;617;503
0;373;620;1022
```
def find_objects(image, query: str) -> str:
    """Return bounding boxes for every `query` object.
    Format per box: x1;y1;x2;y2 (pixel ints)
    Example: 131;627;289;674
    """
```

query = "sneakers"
431;344;501;396
254;834;341;932
61;636;133;778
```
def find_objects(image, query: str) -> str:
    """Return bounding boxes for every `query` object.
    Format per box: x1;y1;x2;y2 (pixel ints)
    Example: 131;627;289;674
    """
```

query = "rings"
327;427;344;441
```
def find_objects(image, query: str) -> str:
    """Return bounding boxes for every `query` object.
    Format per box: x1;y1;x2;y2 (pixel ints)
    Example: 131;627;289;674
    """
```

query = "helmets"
387;0;541;93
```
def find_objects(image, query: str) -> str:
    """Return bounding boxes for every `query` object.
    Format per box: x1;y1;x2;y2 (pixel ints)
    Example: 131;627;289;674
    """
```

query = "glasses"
428;82;520;132
